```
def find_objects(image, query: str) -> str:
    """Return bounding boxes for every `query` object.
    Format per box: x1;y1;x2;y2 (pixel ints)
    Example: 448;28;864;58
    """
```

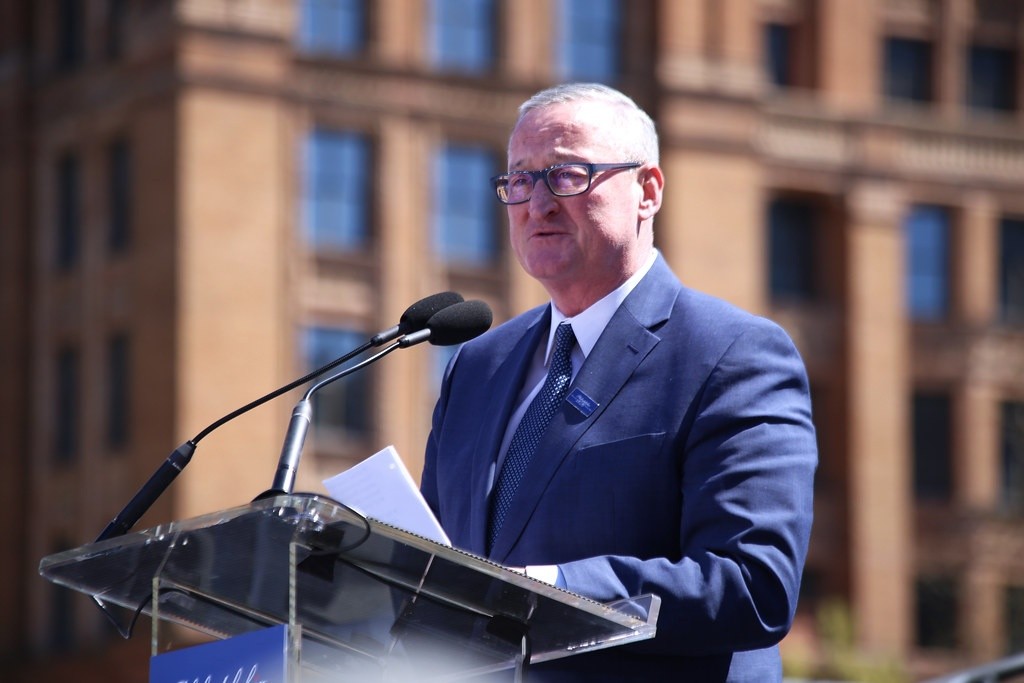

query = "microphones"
96;292;493;557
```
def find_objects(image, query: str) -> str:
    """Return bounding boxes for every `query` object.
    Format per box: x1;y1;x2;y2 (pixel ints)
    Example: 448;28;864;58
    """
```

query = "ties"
486;321;577;557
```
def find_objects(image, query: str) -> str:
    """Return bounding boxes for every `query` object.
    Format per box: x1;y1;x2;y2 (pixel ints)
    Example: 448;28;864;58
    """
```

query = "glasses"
490;162;644;204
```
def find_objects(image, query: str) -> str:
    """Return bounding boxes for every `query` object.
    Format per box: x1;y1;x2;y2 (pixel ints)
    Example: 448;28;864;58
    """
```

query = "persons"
415;80;819;683
